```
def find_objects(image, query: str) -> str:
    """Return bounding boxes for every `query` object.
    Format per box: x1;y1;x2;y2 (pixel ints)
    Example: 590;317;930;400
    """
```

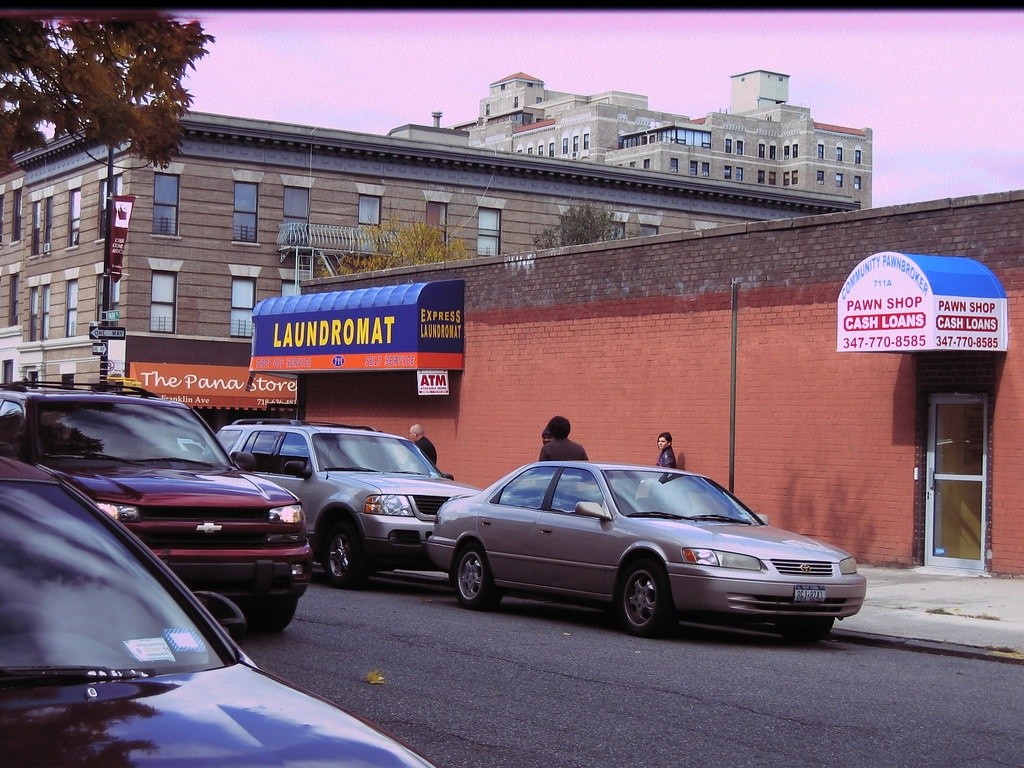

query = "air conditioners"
44;243;51;252
570;152;579;158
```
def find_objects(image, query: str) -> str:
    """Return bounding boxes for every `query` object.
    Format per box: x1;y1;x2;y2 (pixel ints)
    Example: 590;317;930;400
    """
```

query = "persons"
409;423;437;466
655;431;676;469
539;415;589;463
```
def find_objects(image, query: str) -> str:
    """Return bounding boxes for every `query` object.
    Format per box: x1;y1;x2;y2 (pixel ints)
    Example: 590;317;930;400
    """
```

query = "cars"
1;460;435;766
424;460;867;643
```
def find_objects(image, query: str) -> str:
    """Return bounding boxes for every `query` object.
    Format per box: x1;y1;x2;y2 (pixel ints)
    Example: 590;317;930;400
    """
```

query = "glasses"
542;436;552;439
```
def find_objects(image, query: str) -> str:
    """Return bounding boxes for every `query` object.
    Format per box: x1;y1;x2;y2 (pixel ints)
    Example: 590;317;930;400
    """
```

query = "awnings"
835;252;1007;355
129;362;296;411
248;278;466;380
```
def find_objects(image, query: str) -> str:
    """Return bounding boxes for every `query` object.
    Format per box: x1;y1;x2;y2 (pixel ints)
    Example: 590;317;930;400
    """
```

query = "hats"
542;427;553;437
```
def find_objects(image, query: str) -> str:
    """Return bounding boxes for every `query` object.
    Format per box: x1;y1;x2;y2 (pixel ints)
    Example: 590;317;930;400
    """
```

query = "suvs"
203;419;486;586
1;377;313;632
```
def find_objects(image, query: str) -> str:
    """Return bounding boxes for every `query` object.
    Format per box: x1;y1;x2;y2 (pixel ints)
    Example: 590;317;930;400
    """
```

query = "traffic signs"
91;342;107;356
88;325;125;341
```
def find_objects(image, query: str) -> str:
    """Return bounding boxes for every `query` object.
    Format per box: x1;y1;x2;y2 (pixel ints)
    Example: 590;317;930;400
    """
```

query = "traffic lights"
115;380;132;392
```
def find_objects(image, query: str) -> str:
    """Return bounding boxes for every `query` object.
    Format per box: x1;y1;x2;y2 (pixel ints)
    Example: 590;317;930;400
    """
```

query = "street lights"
53;92;171;386
